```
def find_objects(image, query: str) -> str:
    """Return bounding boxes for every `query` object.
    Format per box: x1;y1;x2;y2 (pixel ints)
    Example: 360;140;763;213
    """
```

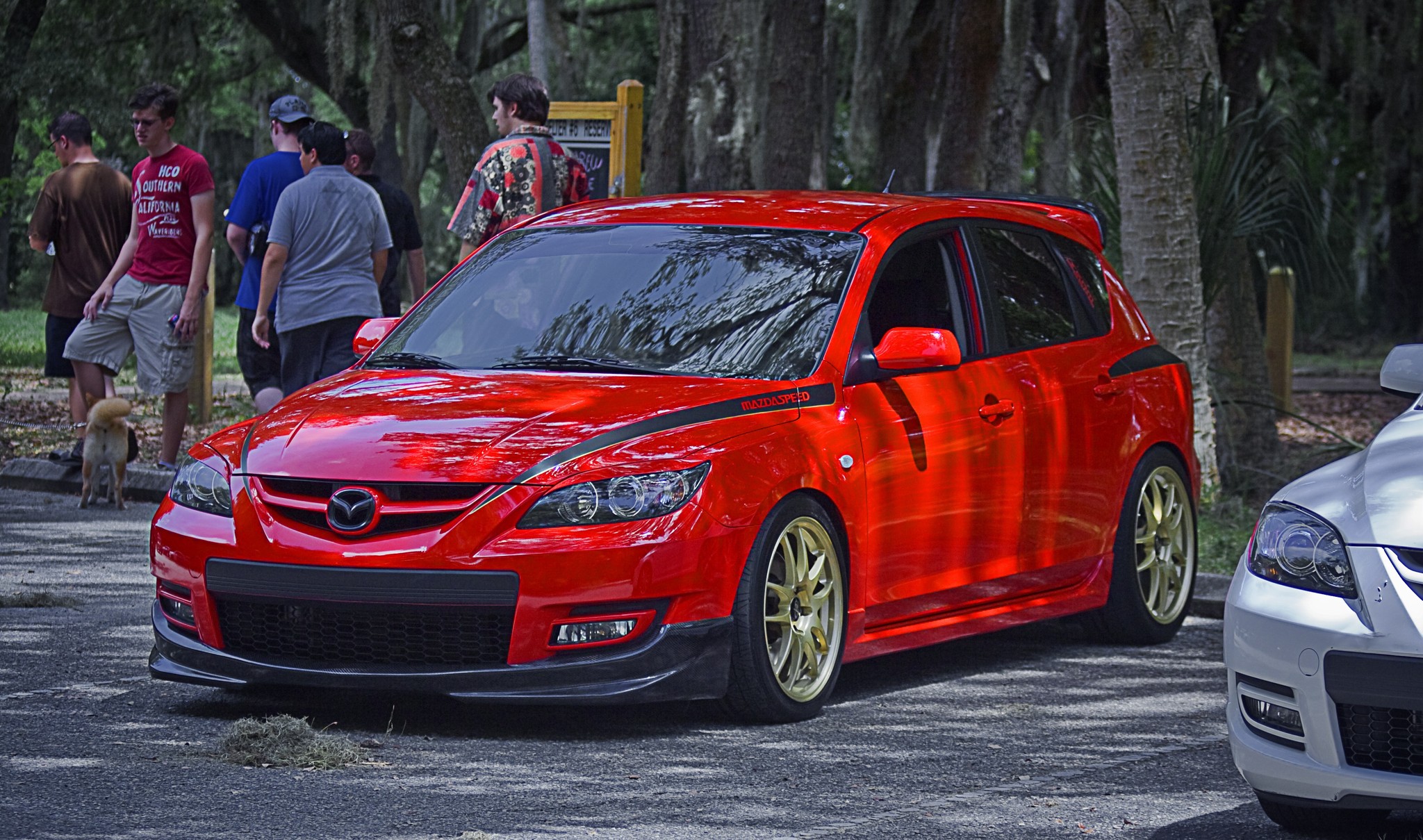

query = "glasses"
343;130;357;154
307;122;317;137
47;134;68;152
129;113;174;128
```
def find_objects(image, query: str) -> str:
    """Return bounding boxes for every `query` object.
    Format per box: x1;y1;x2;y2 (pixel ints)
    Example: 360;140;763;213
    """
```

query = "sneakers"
48;438;85;466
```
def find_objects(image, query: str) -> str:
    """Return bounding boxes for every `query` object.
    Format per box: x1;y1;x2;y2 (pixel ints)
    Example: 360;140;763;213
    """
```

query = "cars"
1221;344;1422;835
147;190;1205;725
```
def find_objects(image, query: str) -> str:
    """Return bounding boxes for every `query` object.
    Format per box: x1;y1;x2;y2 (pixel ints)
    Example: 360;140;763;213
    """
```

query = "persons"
448;72;592;352
225;95;428;417
62;86;215;468
28;108;143;465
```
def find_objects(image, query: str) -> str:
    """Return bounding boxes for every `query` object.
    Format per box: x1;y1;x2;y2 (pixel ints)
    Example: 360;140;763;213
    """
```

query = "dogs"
77;392;130;511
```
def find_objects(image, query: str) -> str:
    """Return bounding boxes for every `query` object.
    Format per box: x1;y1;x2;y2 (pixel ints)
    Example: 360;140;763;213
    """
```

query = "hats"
269;95;315;124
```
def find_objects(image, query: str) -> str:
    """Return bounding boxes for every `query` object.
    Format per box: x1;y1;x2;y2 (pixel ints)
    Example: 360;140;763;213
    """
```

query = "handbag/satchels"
223;220;269;256
